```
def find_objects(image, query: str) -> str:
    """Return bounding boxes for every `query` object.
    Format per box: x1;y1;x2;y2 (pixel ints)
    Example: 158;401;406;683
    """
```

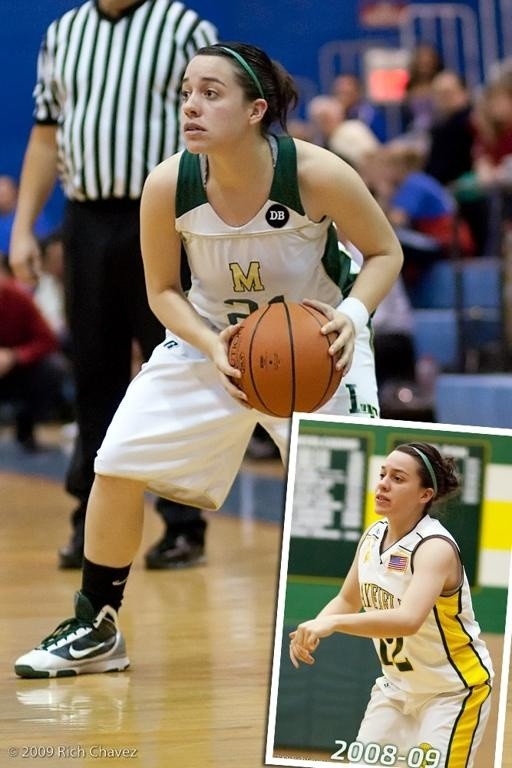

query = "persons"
7;2;228;571
287;439;497;765
11;40;408;679
0;40;512;458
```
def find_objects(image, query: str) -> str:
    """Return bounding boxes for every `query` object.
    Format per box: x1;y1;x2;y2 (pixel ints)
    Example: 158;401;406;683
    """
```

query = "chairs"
377;192;512;430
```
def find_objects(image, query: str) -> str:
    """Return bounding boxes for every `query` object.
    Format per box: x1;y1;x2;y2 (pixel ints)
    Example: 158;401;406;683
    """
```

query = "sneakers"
146;529;205;569
14;590;129;677
57;527;83;568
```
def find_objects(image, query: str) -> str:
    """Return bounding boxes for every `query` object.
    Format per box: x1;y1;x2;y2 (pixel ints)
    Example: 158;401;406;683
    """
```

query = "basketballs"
229;301;344;417
